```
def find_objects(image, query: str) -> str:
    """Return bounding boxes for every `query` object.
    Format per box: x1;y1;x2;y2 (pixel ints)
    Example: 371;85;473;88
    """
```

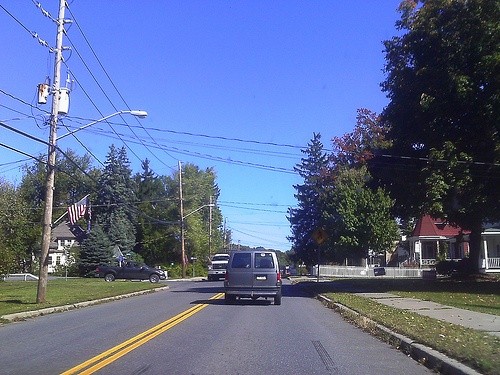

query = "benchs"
423;270;436;279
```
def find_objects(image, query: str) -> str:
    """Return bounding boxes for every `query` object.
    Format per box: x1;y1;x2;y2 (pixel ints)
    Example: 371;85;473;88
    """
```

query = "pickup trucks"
93;260;166;284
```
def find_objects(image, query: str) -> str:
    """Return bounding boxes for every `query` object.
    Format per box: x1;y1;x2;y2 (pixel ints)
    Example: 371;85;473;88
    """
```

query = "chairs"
260;259;271;268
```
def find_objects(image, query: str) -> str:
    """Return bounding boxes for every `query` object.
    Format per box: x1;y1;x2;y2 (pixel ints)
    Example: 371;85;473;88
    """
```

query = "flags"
68;198;86;225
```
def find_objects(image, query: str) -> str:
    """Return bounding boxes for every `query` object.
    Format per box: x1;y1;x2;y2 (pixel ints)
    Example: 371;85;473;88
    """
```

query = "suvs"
207;253;229;281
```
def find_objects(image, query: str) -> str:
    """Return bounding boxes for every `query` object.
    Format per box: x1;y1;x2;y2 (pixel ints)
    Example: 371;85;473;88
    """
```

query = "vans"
223;250;282;306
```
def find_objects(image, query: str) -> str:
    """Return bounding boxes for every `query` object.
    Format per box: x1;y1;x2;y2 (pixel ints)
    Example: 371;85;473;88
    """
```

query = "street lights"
34;108;148;301
180;203;215;278
